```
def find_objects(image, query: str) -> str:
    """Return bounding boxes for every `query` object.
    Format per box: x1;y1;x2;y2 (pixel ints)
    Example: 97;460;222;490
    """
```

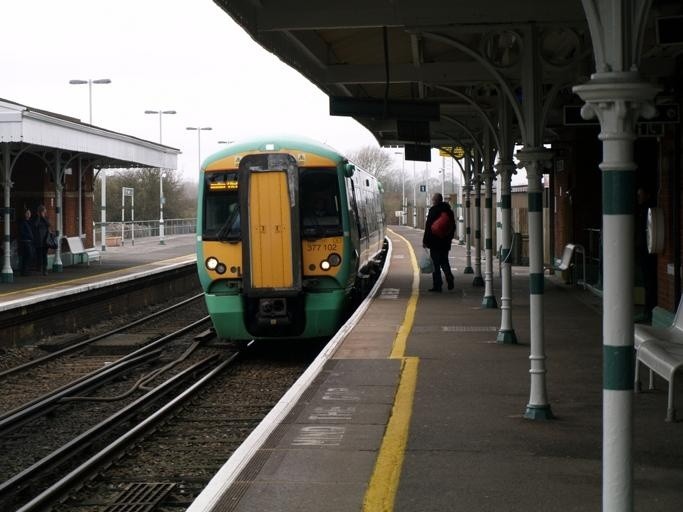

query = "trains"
195;135;388;352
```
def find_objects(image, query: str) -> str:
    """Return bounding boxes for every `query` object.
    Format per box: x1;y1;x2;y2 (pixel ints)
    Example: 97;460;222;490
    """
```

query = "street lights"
186;127;234;181
69;78;111;126
145;109;177;245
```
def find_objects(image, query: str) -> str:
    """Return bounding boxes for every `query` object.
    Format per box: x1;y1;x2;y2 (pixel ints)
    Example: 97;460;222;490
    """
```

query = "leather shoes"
428;285;441;293
446;274;454;290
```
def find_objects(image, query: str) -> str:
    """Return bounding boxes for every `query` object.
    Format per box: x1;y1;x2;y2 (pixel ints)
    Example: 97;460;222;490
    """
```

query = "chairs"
635;290;683;422
542;244;586;295
62;237;101;268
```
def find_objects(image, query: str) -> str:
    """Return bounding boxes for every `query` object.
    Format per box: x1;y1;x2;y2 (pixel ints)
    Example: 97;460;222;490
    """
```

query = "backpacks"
430;211;450;239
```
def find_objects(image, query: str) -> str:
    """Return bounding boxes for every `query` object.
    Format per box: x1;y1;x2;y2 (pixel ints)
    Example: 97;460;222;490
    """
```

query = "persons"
422;193;456;292
18;207;34;277
32;206;51;276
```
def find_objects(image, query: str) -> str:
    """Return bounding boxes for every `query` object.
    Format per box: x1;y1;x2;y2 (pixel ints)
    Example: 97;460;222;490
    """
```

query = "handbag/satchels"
48;238;57;249
